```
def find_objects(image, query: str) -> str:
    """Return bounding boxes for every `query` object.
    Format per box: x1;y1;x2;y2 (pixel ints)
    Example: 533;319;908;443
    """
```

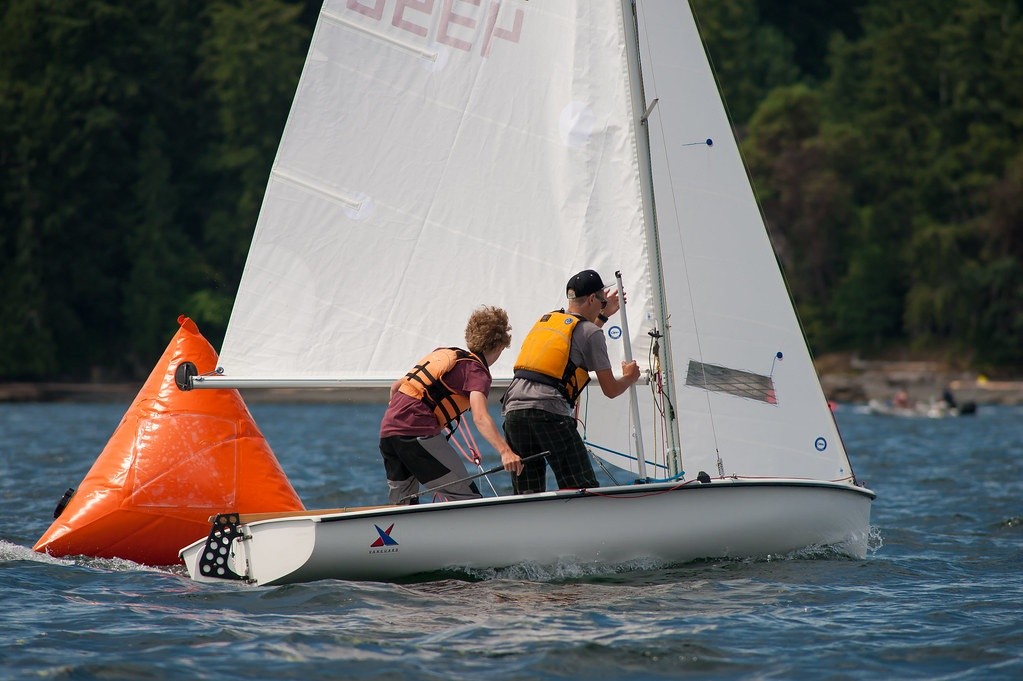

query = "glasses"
593;293;608;309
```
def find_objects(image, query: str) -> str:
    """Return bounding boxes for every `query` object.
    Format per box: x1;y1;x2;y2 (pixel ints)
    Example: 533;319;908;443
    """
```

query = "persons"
363;304;524;505
499;270;641;495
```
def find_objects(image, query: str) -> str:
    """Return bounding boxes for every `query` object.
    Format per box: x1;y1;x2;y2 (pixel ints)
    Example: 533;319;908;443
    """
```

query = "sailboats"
174;1;878;589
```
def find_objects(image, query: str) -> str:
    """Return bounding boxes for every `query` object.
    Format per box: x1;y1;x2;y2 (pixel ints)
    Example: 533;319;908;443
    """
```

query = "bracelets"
598;313;608;323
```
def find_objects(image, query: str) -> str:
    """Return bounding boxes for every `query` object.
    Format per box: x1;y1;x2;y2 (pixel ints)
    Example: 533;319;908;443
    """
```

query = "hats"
567;270;617;299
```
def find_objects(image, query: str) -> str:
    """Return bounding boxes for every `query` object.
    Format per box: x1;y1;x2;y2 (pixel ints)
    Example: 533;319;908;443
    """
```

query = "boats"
867;398;921;419
